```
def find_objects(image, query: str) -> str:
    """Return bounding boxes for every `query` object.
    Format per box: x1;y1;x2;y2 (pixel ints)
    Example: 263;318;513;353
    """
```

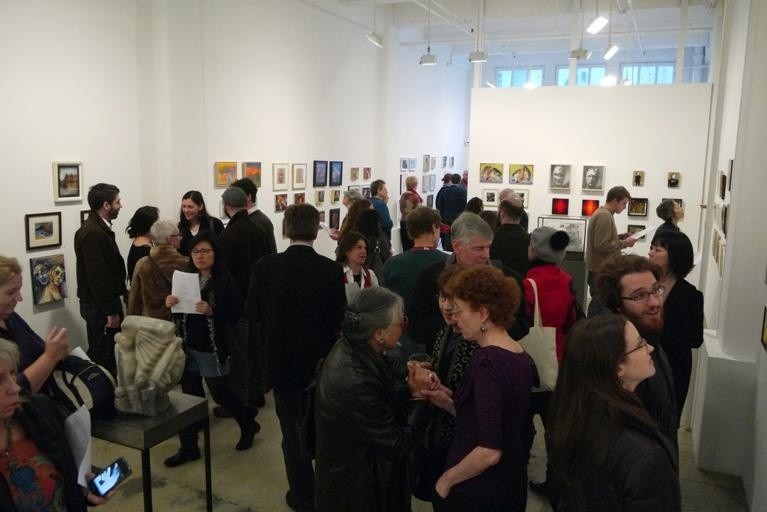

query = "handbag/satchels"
374;246;383;280
515;326;558;396
47;354;117;417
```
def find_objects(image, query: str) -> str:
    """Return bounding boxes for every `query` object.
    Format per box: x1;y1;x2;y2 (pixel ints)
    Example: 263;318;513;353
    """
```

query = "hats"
221;187;247;207
530;225;569;266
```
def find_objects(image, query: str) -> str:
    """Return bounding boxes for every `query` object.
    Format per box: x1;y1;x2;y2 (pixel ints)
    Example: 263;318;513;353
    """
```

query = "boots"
164;440;201;467
235;417;261;450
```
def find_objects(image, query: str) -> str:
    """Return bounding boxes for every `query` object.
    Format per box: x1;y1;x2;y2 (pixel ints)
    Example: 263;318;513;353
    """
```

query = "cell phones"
88;457;132;497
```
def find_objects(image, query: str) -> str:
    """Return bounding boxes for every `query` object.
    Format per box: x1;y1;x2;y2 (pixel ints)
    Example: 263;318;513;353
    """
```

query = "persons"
0;170;703;512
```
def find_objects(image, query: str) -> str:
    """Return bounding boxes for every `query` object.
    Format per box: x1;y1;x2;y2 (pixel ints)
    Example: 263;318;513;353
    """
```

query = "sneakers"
213;406;232;419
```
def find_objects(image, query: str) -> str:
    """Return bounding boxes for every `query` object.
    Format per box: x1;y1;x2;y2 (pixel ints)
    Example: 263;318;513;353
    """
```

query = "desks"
90;390;212;512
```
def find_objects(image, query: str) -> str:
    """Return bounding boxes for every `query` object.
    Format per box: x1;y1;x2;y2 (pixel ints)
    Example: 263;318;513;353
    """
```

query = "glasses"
391;316;409;331
435;292;453;303
621;283;665;304
190;248;215;254
451;304;465;319
169;230;184;237
621;338;649;360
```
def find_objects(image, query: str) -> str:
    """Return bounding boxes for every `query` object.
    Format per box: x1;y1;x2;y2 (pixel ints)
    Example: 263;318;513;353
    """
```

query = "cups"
407;351;431;364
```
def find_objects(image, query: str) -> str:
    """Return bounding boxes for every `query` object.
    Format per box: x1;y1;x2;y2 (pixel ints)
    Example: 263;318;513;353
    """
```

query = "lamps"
569;0;592;61
585;0;608;35
603;0;619;59
469;0;488;63
365;0;384;49
419;0;438;67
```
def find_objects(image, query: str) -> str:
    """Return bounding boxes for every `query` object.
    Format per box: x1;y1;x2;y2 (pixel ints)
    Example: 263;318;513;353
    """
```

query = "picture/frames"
51;160;82;202
81;209;93;226
399;153;454;209
481;189;499;208
628;198;647;217
273;160;372;230
511;189;528;210
25;212;62;251
628;225;645;238
